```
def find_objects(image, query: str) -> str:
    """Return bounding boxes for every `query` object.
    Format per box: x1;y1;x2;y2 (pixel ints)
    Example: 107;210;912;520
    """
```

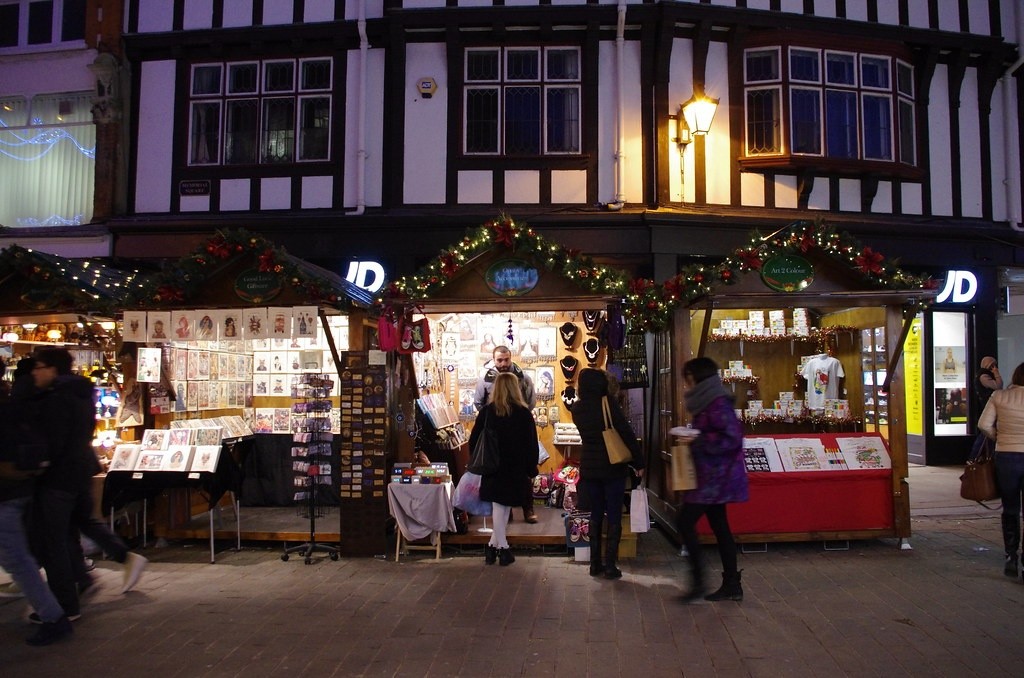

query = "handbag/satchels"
959;436;1000;501
378;306;397;352
452;471;492;516
465;406;489;475
670;439;698;491
398;303;431;354
602;396;636;464
630;477;650;532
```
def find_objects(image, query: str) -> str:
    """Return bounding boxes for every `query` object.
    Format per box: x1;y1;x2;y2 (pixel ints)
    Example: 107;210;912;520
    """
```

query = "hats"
981;356;996;369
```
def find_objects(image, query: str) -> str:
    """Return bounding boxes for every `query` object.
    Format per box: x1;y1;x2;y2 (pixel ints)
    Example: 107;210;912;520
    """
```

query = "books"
418;391;458;427
107;425;223;472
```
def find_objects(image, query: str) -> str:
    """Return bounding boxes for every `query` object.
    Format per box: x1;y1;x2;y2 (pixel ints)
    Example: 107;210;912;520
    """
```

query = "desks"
387;482;452;562
102;468;241;564
554;437;583;459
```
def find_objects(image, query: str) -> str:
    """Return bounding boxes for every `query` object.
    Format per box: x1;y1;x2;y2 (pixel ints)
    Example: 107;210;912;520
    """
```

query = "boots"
704;569;743;600
605;525;621;579
1001;513;1020;577
680;569;704;603
588;522;605;575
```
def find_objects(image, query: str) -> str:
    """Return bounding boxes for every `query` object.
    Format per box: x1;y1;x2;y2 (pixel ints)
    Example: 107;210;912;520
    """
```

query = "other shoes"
0;582;27;597
486;544;496;564
29;605;81;624
507;511;513;522
77;574;98;599
25;615;73;645
500;547;515;565
524;511;538;523
120;551;147;594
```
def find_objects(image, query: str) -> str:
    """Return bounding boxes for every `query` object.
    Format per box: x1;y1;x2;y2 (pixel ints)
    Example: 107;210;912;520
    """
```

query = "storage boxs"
601;514;638;539
734;391;850;419
711;307;815;336
716;360;753;379
601;540;637;556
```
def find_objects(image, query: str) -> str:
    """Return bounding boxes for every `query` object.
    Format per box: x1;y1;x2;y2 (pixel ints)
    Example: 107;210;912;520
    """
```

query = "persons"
967;356;1003;465
943;347;957;373
976;362;1024;582
0;347;150;646
468;372;539;567
672;357;750;600
473;345;538;530
569;367;644;579
799;353;844;411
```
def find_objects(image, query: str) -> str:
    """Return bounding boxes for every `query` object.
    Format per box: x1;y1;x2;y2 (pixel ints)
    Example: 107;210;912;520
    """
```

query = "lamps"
672;79;721;144
22;321;38;333
3;101;15;112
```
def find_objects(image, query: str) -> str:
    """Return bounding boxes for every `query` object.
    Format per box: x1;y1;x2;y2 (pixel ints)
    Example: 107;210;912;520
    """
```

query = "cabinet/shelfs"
708;327;859;382
0;339;121;389
